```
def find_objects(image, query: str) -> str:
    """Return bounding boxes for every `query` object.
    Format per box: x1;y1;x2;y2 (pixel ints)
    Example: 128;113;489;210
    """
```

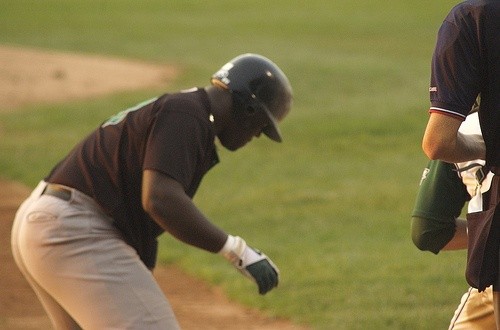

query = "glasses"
261;118;273;133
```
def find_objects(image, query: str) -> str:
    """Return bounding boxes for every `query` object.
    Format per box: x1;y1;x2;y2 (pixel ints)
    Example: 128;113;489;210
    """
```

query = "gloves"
214;233;281;296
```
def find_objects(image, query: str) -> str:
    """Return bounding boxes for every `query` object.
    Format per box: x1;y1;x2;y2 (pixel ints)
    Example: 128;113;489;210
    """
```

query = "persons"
411;94;499;330
10;53;294;330
423;1;498;330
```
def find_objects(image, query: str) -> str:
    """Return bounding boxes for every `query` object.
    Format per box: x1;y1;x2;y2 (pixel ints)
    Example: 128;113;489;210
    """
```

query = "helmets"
210;53;294;143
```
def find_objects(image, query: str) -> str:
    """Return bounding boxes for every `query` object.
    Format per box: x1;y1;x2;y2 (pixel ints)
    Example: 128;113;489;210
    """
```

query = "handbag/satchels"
114;220;159;269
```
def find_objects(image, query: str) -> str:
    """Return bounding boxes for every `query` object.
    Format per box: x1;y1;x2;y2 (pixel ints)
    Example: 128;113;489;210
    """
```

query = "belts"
42;184;72;201
